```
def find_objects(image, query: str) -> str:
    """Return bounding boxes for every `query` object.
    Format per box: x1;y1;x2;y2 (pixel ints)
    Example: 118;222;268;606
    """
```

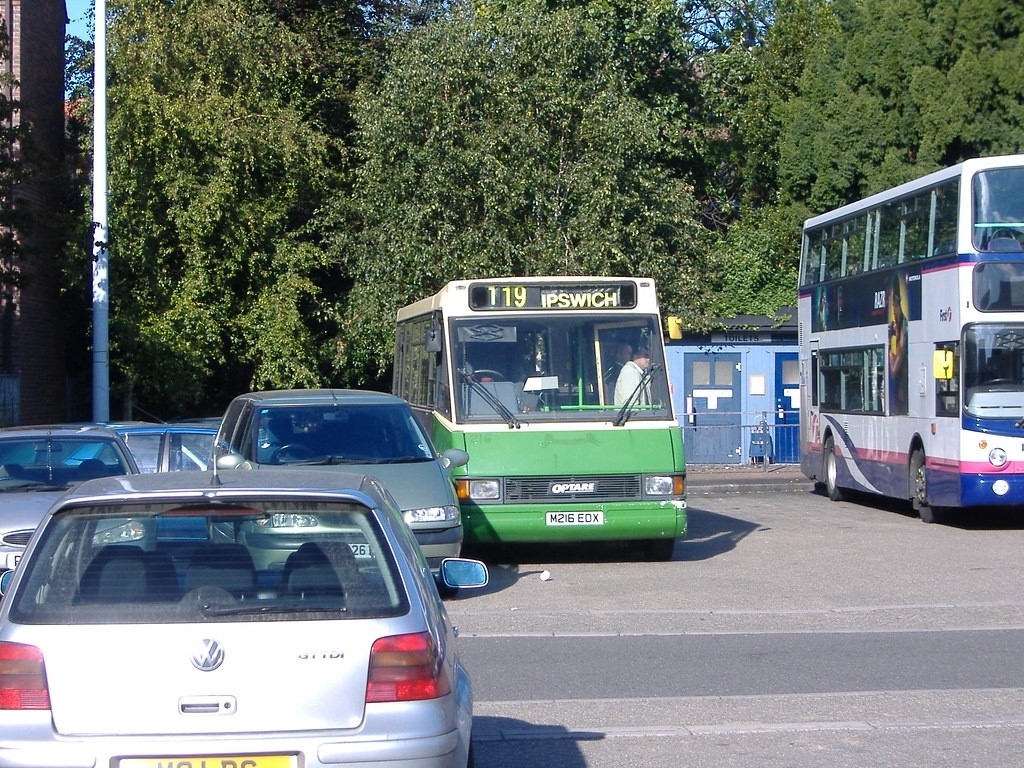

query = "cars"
95;419;227;473
1;473;489;768
1;432;145;607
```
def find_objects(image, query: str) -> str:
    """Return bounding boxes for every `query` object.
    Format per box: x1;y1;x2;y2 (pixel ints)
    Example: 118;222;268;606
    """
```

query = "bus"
391;277;688;564
796;154;1024;526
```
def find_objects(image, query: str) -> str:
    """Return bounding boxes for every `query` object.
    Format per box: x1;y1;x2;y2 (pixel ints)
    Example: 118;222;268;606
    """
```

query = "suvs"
216;389;471;602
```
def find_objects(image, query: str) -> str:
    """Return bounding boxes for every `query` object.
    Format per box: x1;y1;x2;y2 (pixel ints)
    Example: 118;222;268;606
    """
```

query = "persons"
614;346;651;411
604;344;632;405
888;274;909;415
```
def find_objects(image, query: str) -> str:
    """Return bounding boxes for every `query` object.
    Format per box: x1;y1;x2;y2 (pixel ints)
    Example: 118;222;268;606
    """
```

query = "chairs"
0;464;27;480
807;229;1023;285
75;459;111;481
277;541;366;596
345;413;386;459
72;545;179;604
184;543;260;602
